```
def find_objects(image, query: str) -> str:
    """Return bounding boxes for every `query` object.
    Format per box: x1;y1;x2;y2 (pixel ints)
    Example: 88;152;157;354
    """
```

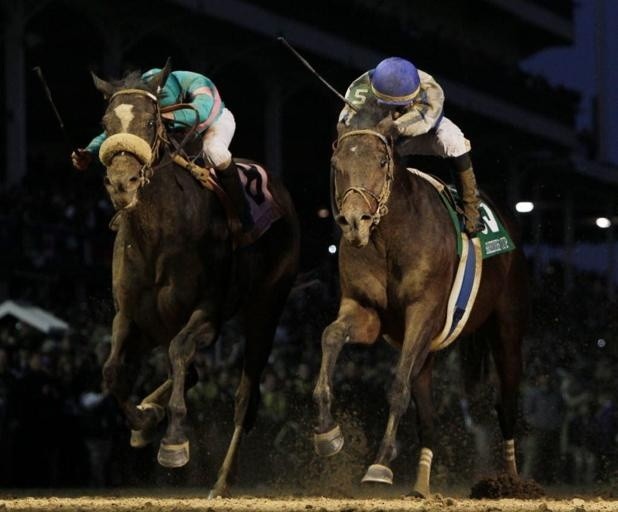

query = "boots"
447;161;481;232
214;158;255;235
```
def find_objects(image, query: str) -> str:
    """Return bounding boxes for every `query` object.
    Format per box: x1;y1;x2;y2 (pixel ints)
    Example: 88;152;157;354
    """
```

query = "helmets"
141;69;181;108
371;57;420;106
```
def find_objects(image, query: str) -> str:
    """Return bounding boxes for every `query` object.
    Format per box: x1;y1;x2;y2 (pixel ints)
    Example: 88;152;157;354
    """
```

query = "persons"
71;69;257;235
333;56;484;237
2;210;617;485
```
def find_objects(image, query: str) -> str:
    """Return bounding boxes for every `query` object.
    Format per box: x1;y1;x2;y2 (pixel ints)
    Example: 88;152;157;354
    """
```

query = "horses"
91;55;306;498
311;110;525;499
